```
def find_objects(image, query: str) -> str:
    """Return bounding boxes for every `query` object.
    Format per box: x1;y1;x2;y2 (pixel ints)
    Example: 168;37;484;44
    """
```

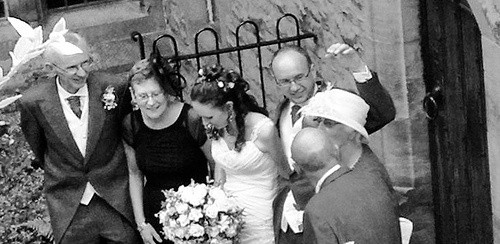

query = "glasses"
53;57;93;75
276;66;310;87
135;92;161;101
314;118;341;128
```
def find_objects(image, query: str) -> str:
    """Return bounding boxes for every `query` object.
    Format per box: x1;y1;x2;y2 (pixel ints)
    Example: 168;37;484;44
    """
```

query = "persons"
19;32;135;244
191;63;293;244
272;43;396;244
299;89;396;202
291;127;403;244
119;45;215;244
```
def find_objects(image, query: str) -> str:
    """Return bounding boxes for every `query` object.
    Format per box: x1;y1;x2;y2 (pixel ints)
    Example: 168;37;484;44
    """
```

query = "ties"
67;96;82;119
291;105;301;127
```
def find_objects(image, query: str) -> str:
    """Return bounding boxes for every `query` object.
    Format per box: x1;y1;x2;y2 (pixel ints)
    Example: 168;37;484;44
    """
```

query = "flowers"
101;84;119;110
154;179;246;244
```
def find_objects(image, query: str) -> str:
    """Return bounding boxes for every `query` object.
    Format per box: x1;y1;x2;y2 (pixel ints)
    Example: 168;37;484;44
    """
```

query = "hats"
297;88;369;140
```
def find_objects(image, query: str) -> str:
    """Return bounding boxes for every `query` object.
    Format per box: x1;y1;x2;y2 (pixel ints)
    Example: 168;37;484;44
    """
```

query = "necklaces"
224;130;238;143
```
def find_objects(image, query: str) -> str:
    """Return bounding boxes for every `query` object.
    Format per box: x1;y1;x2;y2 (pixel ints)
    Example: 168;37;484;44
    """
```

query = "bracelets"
137;223;147;232
